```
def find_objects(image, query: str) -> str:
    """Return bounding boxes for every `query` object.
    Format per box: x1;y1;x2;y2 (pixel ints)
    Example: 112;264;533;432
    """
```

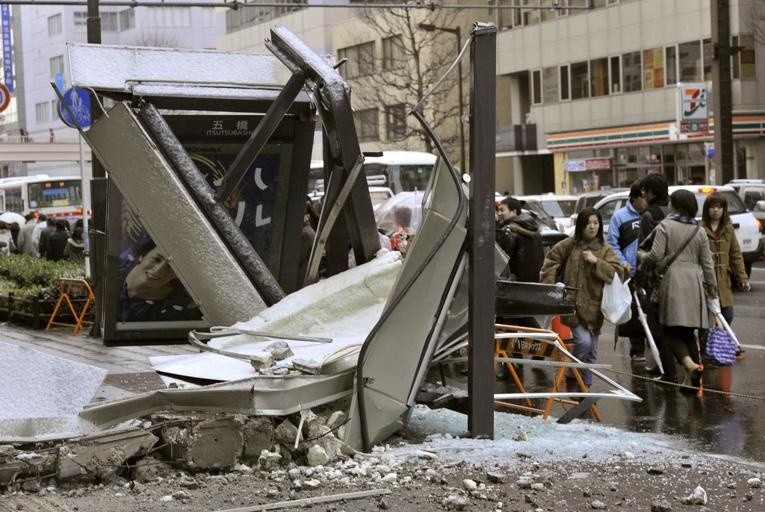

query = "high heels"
679;366;703;393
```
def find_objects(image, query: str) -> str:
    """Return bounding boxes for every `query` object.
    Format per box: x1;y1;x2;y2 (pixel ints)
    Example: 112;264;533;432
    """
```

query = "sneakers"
631;354;646;362
496;371;510;381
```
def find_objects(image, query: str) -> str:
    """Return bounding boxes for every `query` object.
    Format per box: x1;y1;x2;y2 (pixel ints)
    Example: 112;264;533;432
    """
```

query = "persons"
539;208;620;402
117;236;192;321
697;192;751;370
302;192;326;259
0;212;85;261
636;189;720;392
494;198;545;368
348;205;417;269
606;178;671;375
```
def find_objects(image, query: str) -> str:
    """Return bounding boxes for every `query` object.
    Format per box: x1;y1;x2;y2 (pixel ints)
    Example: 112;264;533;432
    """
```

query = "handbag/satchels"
555;267;564;281
618;301;647;338
706;327;736;364
648;273;660;308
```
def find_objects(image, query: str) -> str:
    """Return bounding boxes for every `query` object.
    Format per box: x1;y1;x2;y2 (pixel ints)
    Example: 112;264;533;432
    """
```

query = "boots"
566;376;596;406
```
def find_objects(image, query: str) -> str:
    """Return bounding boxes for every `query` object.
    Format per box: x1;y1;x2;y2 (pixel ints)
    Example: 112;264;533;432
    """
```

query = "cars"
310;151;765;282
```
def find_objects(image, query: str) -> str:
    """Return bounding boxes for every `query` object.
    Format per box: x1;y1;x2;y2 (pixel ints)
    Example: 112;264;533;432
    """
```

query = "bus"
0;174;93;226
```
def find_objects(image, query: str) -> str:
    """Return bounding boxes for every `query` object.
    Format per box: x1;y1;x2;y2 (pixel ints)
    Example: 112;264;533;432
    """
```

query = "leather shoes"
645;366;668;384
